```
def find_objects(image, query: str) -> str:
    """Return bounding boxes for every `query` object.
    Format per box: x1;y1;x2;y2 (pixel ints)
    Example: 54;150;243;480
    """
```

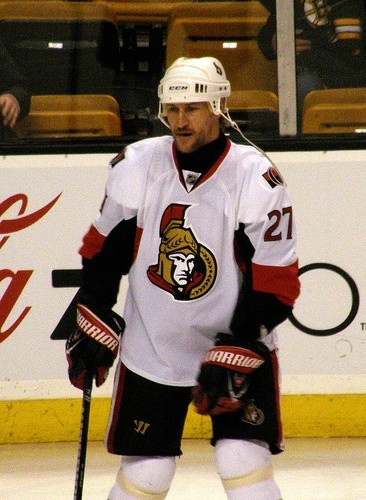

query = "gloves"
63;302;127;388
194;332;268;416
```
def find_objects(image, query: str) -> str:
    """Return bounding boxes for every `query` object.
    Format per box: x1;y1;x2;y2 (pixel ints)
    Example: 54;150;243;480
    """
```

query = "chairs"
2;0;366;145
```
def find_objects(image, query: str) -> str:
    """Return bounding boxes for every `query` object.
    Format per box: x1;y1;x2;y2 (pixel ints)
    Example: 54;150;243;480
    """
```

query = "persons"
0;38;32;156
256;0;366;137
67;55;302;500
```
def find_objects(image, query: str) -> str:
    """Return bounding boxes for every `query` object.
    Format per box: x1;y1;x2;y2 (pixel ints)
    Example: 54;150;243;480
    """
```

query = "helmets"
155;54;289;186
